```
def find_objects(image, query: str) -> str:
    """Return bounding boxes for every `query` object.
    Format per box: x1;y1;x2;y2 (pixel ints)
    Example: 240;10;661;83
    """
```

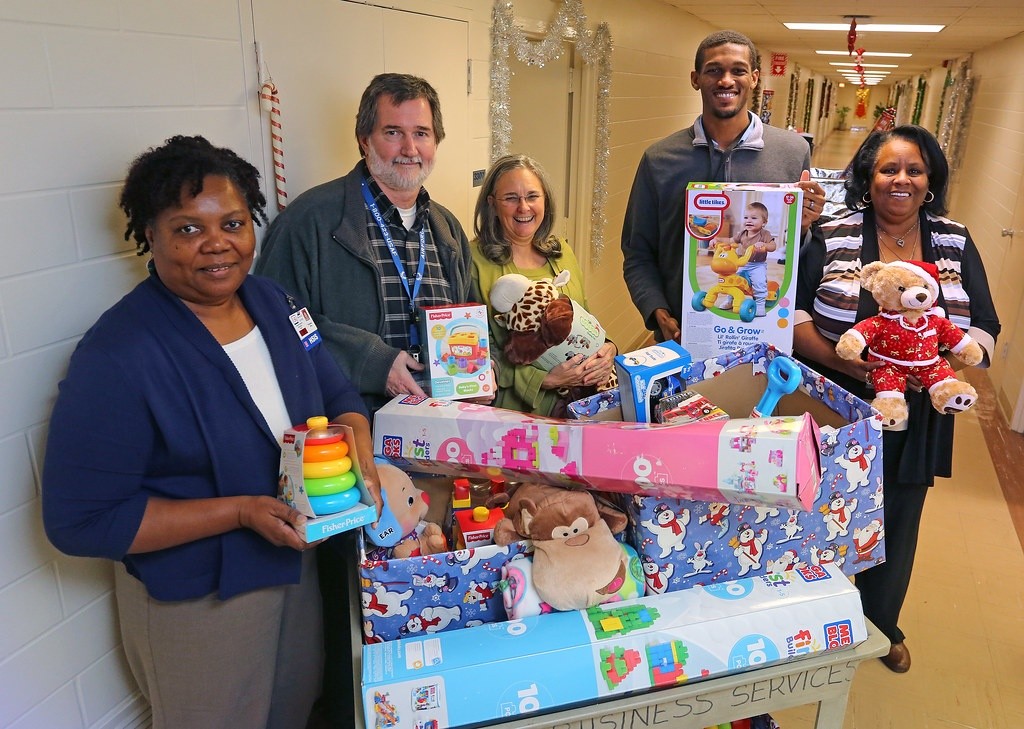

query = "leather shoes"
880;644;910;673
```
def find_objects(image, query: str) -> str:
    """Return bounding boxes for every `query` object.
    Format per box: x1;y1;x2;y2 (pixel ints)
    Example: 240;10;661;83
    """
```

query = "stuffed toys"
370;456;446;559
488;270;606;373
837;261;984;429
486;481;626;612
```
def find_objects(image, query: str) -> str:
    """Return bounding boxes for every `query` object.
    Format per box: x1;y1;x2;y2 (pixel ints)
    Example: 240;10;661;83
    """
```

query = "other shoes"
756;305;766;316
719;300;733;310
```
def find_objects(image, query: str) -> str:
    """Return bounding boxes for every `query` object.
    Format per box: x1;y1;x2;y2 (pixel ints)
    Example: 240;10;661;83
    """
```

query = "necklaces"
877;218;920;264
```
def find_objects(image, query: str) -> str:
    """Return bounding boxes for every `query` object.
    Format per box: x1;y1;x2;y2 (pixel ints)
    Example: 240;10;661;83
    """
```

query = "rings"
602;376;605;381
809;202;814;209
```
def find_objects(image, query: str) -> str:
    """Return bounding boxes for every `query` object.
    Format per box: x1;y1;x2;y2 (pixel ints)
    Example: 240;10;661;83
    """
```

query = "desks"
351;571;891;729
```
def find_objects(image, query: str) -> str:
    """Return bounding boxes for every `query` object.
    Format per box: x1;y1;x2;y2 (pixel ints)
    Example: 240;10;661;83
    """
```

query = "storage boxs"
356;182;887;729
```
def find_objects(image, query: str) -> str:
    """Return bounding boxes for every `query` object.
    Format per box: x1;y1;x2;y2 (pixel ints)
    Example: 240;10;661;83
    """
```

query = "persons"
790;126;1000;673
43;134;383;728
259;73;498;443
277;474;292;499
468;155;618;420
621;34;827;343
707;202;776;316
